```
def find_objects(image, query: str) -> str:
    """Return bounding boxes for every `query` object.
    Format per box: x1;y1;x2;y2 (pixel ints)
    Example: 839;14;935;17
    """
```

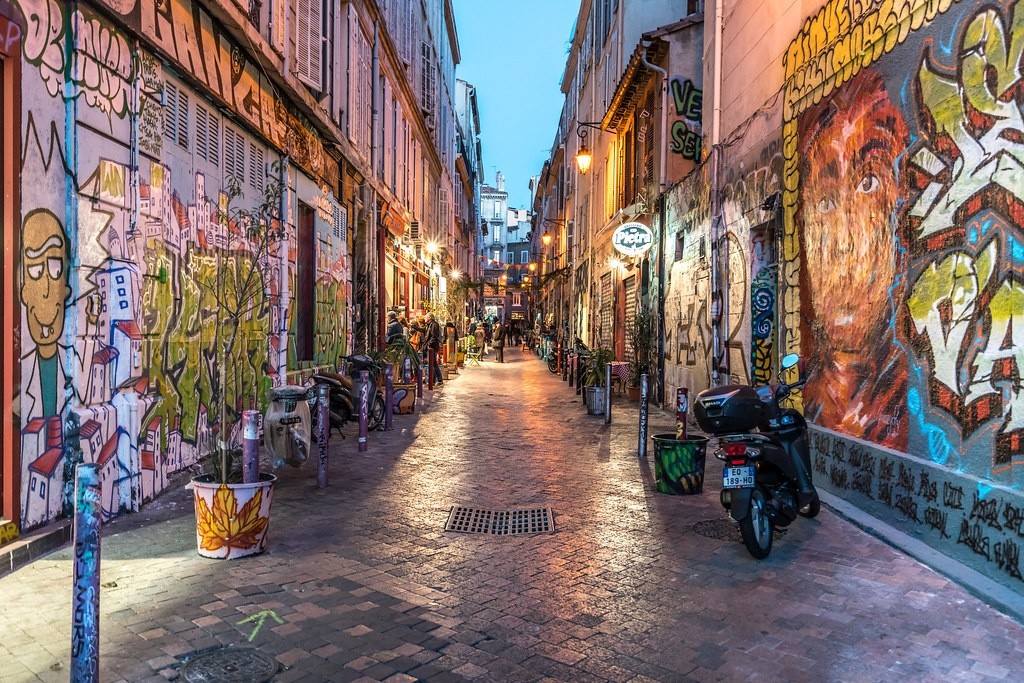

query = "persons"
386;311;443;387
444;318;458;352
466;316;557;363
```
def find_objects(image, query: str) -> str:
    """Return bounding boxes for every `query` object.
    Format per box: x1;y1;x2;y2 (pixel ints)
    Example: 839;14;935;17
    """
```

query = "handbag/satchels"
493;340;503;347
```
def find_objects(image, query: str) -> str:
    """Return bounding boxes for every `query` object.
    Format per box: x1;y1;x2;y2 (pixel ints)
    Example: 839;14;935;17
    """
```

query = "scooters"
303;352;386;444
547;332;595;383
693;352;821;559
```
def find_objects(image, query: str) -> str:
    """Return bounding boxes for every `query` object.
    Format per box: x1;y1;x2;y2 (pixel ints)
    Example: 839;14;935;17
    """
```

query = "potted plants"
627;298;660;401
356;332;423;415
185;146;292;560
575;343;621;418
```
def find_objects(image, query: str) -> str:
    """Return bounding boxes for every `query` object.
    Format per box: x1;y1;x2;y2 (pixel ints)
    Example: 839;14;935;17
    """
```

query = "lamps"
573;120;617;176
520;253;548;288
542;217;565;247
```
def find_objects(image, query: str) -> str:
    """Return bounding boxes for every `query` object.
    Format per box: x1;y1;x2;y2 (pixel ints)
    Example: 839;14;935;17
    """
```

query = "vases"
650;432;710;496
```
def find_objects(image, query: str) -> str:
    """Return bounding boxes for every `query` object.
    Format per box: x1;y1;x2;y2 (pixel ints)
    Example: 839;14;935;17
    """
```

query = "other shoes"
434;382;443;387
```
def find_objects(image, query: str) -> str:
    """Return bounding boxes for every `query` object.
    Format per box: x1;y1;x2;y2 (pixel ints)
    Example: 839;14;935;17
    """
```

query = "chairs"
467;345;484;367
